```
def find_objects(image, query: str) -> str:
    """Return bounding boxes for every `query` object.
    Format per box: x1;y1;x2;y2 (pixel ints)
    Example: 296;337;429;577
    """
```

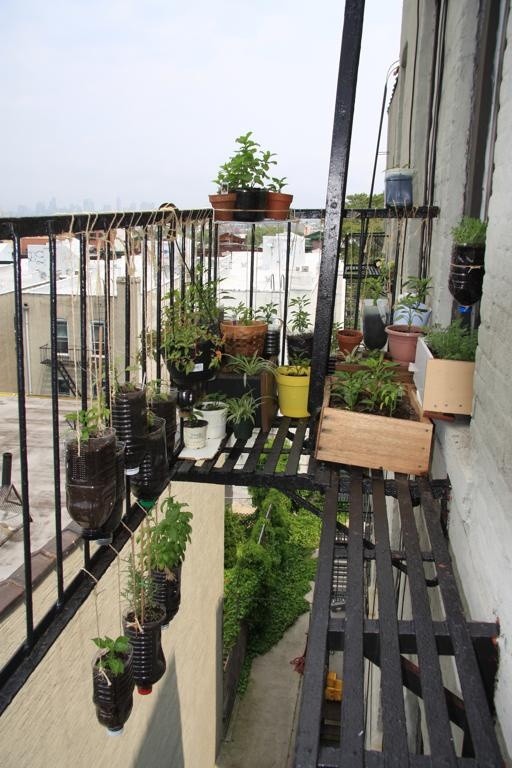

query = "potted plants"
123;256;228;383
79;357;124;549
226;386;279;442
63;404;119;542
135;498;193;631
146;377;179;463
253;301;283;359
282;295;314;365
121;555;164;698
229;130;280;221
103;348;151;480
217;300;269;365
383;163;416;208
182;404;208;449
207;154;243;221
448;210;492;314
192;393;230;441
384;293;428;365
357;259;398;353
392;274;432;326
89;634;138;741
330;315;366;355
265;177;294;221
415;318;479;421
314;346;434;476
273;350;314;419
128;378;170;514
